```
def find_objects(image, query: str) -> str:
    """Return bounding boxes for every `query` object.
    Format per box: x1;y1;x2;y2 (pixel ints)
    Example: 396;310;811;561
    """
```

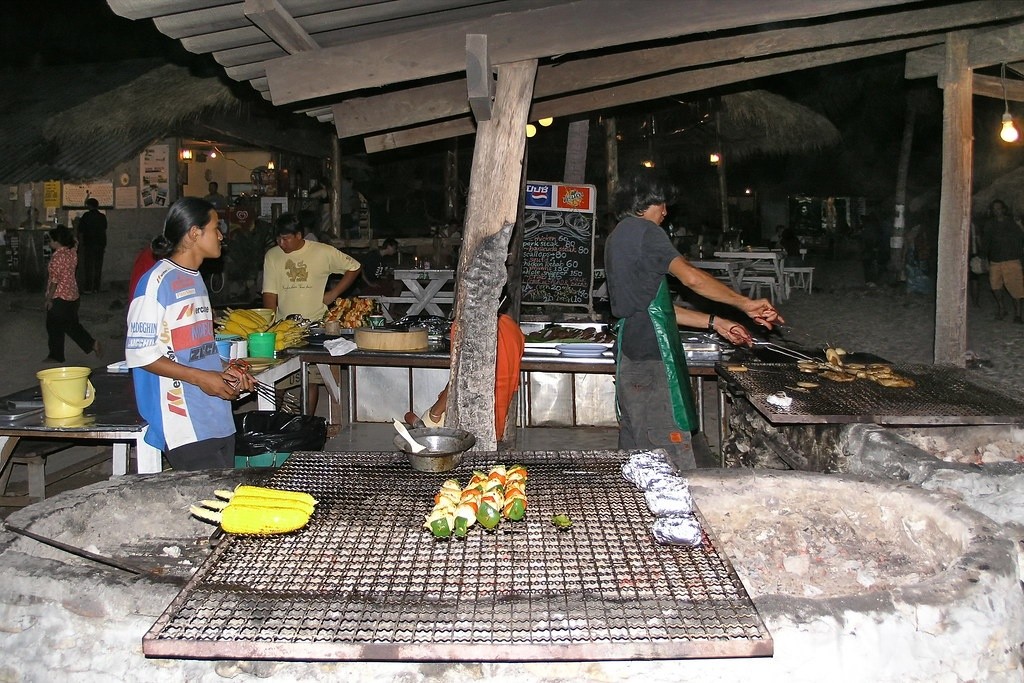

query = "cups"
368;316;384;327
325;320;341;335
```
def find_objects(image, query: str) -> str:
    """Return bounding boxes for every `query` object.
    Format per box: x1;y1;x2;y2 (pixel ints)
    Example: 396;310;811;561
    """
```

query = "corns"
221;486;316;534
220;309;299;351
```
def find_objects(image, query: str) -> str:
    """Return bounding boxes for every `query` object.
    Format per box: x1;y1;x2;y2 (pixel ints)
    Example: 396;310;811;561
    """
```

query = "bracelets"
707;313;716;334
429;412;441;418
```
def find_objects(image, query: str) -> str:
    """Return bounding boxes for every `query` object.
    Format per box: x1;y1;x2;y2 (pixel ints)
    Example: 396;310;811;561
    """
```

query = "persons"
202;182;226;210
438;220;462;269
260;212;364;418
361;237;400;321
420;260;526;452
20;206;44;229
603;167;787;476
40;223;104;366
124;195;259;473
77;197;108;296
127;174;400;338
770;224;806;289
968;198;1024;325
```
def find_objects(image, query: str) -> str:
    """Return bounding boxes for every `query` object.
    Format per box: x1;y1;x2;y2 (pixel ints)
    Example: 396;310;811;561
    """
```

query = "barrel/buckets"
245;332;276;358
250;308;275;327
35;366;96;419
214;340;232;363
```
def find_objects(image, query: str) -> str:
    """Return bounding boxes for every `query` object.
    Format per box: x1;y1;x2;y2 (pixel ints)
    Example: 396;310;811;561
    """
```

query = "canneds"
301;189;308;198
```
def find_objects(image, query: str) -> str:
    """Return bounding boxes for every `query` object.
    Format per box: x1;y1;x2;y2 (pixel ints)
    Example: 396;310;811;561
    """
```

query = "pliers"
723;323;827;368
749;306;855;356
219;361;303;416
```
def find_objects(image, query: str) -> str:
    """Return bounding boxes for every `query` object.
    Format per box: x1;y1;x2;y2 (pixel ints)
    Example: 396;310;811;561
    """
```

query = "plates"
240;357;276;370
555;345;608;354
302;335;349;346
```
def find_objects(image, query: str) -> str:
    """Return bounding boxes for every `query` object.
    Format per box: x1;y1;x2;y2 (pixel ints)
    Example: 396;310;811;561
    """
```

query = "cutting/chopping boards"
354;327;428;350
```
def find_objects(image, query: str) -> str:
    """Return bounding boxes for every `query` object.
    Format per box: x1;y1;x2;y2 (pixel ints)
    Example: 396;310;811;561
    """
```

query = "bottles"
699;245;704;262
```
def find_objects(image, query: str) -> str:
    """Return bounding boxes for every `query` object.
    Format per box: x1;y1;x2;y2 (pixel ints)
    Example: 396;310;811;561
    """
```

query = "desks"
688;260;750;296
393;268;454;316
0;354;301;475
713;250;789;299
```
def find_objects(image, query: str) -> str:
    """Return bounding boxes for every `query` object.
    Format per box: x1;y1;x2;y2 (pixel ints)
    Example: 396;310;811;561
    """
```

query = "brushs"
391;416;433;455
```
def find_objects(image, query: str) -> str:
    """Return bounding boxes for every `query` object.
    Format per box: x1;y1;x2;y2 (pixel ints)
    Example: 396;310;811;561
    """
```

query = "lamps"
183;149;193;160
267;156;274;170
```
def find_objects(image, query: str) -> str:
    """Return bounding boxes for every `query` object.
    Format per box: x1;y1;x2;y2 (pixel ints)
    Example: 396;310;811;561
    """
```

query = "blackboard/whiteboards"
520;180;598;307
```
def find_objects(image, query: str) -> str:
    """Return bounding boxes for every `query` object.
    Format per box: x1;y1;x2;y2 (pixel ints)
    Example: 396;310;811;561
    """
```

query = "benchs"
704;265;819;306
0;437;113;509
355;291;460;304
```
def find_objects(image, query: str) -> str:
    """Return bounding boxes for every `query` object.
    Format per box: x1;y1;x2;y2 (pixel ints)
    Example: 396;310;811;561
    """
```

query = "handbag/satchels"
970;255;989;273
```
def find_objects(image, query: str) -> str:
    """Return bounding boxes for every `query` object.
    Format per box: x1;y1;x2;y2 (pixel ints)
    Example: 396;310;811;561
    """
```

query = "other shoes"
42;356;65;364
93;340;102;358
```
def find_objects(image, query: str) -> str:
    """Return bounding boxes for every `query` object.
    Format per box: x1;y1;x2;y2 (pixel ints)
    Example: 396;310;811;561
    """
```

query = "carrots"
435;469;529;515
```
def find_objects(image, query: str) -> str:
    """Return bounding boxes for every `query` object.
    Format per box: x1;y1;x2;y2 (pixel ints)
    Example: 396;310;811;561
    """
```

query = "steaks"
798;362;915;387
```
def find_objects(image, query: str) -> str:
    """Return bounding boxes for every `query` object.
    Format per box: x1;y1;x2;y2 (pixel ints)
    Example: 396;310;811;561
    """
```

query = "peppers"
430;498;572;537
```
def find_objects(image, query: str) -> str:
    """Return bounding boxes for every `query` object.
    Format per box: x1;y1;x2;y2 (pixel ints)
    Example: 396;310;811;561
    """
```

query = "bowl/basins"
394;427;476;472
